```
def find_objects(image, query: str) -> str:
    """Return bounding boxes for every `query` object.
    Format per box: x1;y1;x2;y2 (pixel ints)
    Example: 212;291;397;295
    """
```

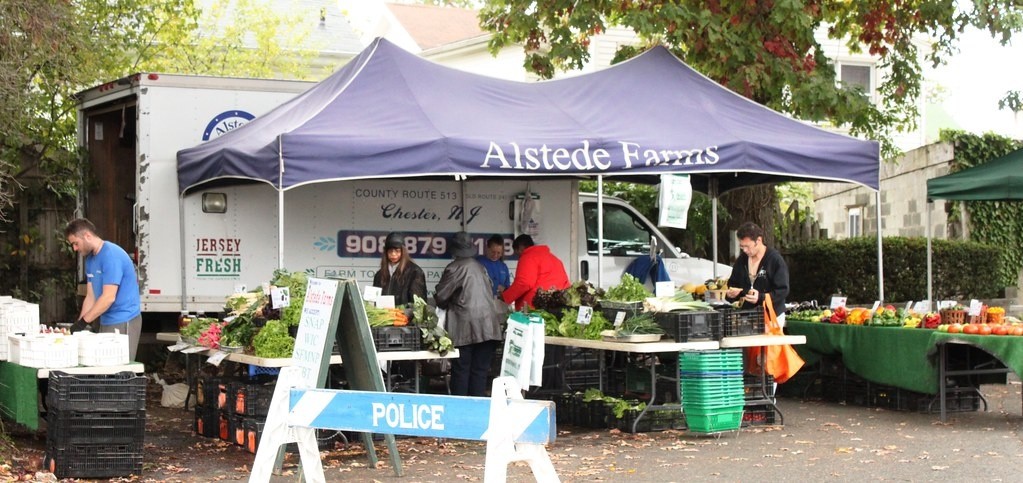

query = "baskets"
987;312;1006;324
44;408;145;445
939;309;966;325
964;309;988;323
0;294;135;369
521;341;746;436
717;307;765;336
44;438;144;476
744;375;775;399
45;370;147;414
744;401;774;427
190;313;437;454
648;309;724;343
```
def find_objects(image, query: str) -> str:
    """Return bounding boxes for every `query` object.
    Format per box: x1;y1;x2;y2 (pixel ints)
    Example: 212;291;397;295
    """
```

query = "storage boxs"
40;361;152;480
1;293;134;374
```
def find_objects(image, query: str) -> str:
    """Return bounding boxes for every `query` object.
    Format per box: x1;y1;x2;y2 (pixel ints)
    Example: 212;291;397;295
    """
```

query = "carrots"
366;304;408;331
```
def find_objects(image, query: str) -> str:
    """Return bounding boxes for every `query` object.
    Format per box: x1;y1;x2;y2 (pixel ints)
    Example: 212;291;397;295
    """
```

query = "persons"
432;230;503;398
371;231;428;314
474;234;511;301
63;216;146;362
726;220;789;334
498;231;572;311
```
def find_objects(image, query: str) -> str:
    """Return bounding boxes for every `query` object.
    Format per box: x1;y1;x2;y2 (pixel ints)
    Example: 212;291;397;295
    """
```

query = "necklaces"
95;238;103;256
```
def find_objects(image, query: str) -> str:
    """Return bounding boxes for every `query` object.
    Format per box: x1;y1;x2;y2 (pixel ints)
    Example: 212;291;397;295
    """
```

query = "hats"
383;231;405;248
448;232;479;257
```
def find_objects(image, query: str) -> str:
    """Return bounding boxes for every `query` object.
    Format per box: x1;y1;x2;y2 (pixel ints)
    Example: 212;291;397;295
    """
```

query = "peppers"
829;306;908;326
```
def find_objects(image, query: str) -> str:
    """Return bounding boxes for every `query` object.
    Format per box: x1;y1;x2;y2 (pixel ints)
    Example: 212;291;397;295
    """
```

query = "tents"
171;32;888;317
926;146;1022;308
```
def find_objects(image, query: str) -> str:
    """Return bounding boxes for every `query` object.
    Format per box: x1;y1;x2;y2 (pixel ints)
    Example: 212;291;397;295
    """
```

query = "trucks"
74;75;736;386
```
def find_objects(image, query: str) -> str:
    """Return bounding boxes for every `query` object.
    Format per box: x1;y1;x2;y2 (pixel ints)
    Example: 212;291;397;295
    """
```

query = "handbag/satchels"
749;294;804;383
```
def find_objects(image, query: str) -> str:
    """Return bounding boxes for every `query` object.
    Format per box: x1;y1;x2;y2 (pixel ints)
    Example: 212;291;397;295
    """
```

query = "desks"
790;313;1023;420
542;332;718;436
717;332;809;426
163;328;460;466
2;360;154;433
